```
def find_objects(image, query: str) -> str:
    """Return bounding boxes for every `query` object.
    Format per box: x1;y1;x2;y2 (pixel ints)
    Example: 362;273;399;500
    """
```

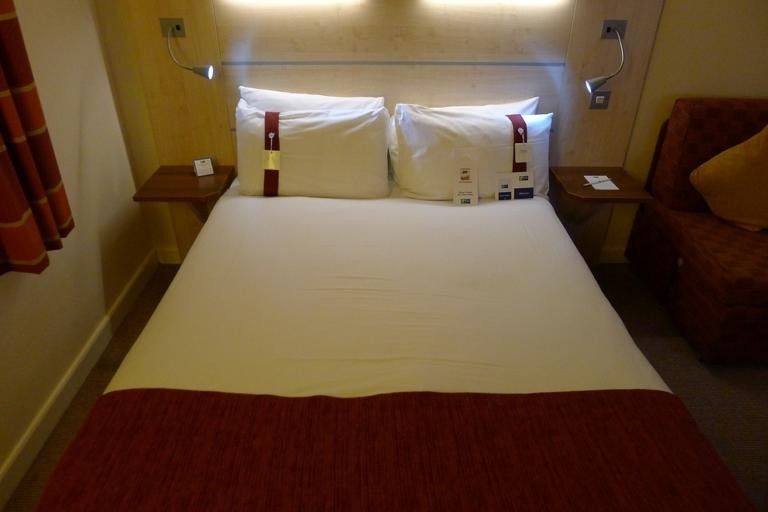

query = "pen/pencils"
583;177;613;187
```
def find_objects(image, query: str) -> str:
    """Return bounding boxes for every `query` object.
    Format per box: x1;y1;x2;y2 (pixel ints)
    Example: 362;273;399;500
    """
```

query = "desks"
133;166;233;224
549;166;652;203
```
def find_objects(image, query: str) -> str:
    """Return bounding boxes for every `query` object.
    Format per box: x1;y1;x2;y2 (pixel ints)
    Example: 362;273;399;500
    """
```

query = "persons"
460;170;470;181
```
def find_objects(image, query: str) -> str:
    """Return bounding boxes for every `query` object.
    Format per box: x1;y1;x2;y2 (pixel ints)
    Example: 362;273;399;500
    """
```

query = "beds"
29;174;762;511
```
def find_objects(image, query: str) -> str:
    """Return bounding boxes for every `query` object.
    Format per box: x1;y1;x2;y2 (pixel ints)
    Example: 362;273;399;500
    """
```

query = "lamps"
584;19;628;95
159;17;214;82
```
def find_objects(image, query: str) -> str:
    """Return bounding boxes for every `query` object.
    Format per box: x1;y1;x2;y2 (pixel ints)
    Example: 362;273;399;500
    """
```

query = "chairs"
626;97;768;364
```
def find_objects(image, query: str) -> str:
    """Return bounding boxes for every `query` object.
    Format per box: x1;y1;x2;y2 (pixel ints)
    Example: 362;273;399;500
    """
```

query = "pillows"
688;125;768;232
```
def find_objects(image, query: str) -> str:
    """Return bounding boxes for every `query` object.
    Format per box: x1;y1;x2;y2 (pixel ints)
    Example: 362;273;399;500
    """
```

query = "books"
583;174;620;191
